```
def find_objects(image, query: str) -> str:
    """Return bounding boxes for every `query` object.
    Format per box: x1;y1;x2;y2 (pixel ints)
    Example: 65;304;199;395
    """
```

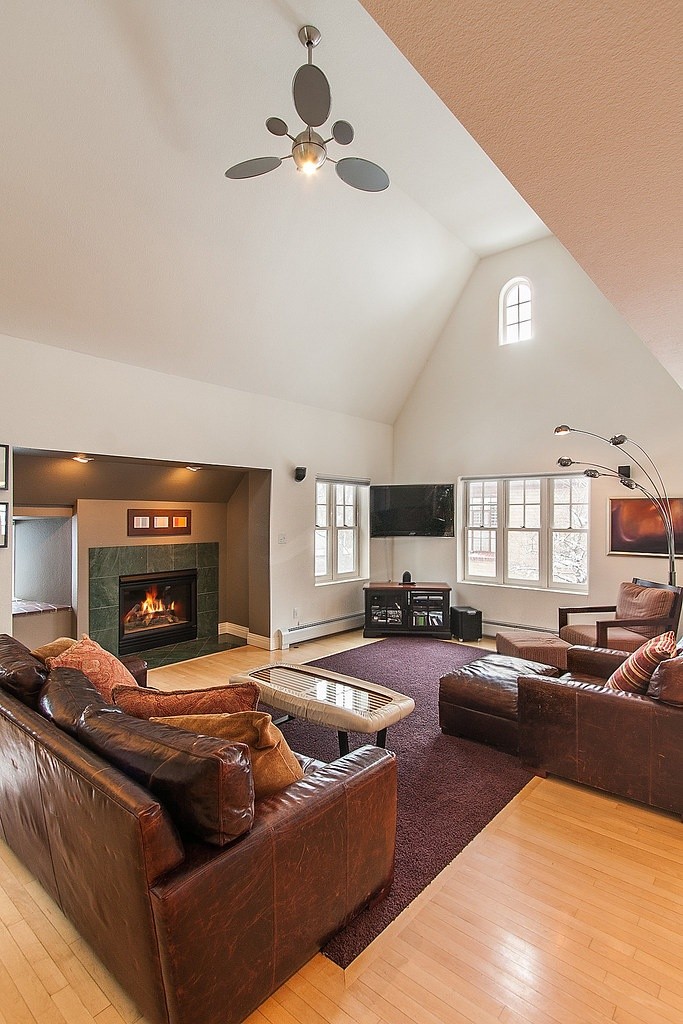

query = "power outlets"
293;608;298;618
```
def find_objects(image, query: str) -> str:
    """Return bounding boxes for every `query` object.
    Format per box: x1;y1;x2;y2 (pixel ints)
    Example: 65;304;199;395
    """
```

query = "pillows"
44;633;139;709
149;709;306;799
110;680;263;721
604;630;677;696
29;637;77;666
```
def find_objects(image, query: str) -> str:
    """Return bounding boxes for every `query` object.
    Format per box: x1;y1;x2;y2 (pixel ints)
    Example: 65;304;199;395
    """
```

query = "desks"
230;660;414;759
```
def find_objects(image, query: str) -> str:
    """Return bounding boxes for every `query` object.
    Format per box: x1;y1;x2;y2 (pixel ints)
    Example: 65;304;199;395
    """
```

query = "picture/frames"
607;496;683;560
0;501;9;548
0;444;9;490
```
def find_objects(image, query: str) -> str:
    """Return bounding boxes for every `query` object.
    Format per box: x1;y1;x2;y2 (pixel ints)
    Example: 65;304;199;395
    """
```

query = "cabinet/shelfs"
362;582;453;640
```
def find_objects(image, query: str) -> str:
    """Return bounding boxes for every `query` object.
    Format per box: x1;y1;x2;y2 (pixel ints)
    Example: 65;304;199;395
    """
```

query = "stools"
439;651;558;755
497;630;572;678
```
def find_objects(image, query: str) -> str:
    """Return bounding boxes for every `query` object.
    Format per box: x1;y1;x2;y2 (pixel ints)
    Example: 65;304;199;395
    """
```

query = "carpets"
258;638;536;970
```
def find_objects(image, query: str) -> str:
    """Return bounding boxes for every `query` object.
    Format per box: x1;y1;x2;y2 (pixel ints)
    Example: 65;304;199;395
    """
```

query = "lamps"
224;24;390;193
553;423;677;586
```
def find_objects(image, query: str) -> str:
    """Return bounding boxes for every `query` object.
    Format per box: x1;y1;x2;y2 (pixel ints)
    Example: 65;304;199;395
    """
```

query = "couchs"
0;633;398;1024
516;644;683;817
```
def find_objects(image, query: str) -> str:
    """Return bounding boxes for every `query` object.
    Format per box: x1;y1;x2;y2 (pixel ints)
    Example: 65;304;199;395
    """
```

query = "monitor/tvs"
370;483;455;538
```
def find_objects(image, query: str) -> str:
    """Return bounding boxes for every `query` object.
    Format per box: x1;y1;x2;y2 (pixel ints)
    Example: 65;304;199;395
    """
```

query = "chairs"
558;577;683;653
